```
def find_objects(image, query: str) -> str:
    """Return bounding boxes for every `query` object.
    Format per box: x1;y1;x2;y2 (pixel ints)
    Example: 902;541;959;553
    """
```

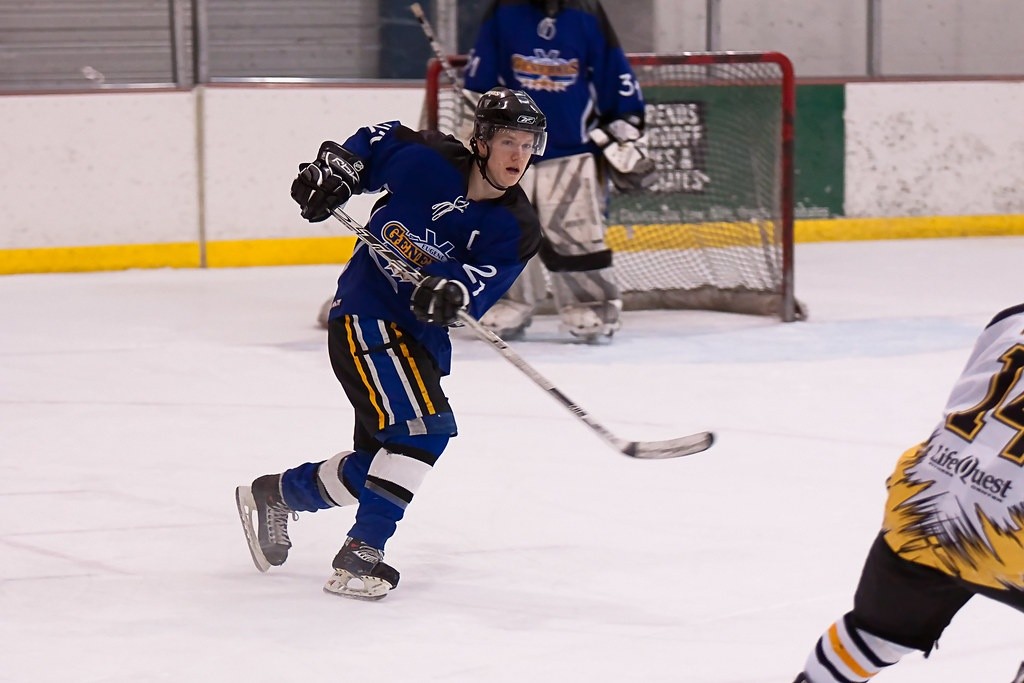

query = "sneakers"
324;535;399;600
236;470;299;573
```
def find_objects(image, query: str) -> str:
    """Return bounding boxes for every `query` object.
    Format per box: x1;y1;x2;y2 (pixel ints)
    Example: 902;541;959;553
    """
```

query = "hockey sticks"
409;2;615;275
332;206;716;461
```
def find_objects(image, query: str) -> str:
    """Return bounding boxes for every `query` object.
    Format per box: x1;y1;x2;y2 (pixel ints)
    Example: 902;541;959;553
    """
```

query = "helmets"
473;88;547;156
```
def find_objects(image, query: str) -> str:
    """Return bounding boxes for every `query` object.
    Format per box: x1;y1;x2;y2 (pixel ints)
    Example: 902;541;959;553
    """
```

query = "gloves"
291;141;369;222
411;276;464;328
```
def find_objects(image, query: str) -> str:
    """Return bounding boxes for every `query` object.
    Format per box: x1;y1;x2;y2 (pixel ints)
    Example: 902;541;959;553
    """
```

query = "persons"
460;0;646;344
236;86;546;603
796;299;1024;681
